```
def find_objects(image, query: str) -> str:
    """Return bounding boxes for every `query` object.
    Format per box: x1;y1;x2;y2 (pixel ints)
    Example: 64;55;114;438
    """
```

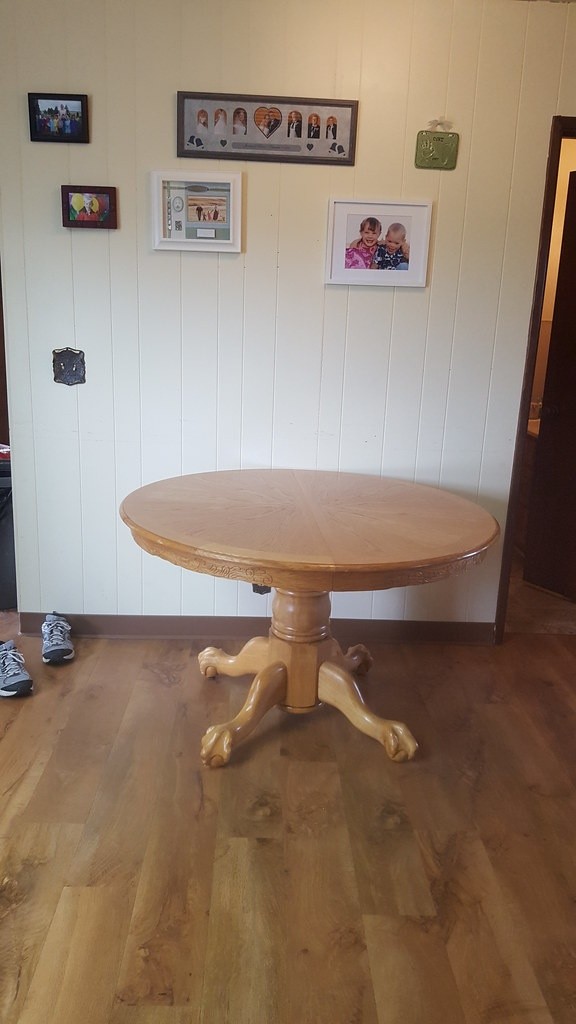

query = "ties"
291;122;295;129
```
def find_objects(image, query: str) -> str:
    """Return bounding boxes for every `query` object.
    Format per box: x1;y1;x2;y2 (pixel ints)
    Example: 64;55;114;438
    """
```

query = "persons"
287;111;302;138
308;114;320;139
215;109;227;136
40;113;79;135
195;110;208;137
345;217;410;270
76;194;99;221
259;111;281;136
196;203;220;222
233;110;247;136
326;117;337;139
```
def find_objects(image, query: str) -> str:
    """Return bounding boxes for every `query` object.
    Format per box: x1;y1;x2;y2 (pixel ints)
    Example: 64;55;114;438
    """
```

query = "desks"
118;468;501;768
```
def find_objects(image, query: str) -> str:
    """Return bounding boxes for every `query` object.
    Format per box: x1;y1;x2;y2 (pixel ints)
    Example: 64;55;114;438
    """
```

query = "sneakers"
41;614;74;664
0;639;33;696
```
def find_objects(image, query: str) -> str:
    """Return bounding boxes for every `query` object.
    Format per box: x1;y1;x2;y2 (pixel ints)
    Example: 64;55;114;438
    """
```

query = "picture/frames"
149;171;242;254
176;90;358;167
61;185;118;229
324;197;433;288
27;92;90;144
